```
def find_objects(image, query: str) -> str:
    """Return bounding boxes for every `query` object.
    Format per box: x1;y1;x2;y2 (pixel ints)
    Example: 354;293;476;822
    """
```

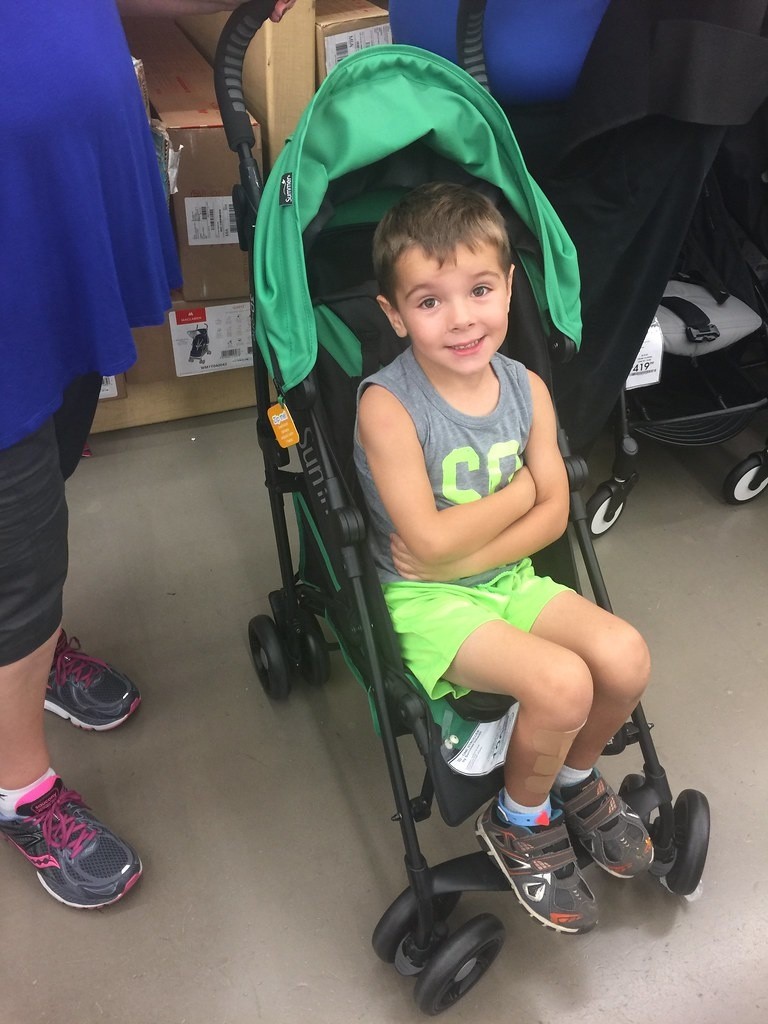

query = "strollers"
208;0;709;1014
526;1;768;538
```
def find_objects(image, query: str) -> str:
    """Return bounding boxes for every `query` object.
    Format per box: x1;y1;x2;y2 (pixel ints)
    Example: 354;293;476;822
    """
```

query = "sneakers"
43;628;142;731
551;767;654;879
475;797;599;935
0;776;142;910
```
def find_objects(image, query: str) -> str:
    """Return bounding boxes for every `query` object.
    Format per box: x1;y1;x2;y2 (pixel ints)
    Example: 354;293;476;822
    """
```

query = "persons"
0;0;182;915
352;181;654;937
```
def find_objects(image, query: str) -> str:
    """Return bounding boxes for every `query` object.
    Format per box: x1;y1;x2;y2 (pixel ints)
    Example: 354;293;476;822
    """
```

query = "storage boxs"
88;17;278;434
314;0;393;89
